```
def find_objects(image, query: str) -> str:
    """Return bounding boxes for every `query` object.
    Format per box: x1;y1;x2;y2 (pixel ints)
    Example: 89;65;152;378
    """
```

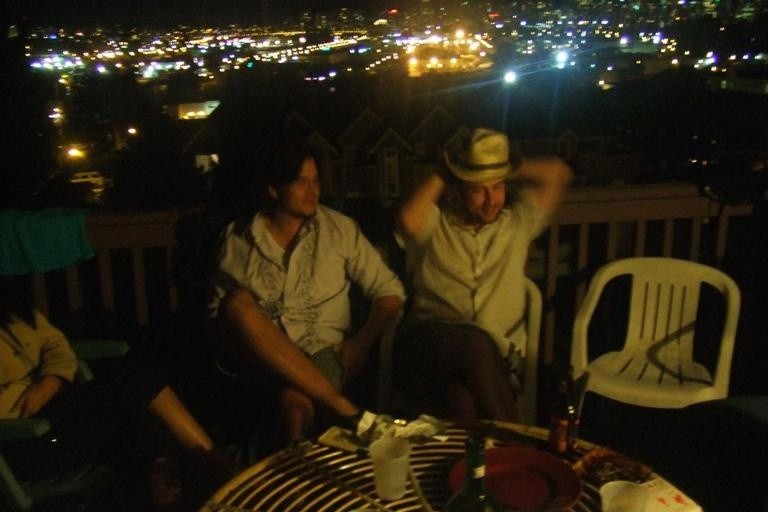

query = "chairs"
378;275;543;425
570;256;743;426
0;457;126;512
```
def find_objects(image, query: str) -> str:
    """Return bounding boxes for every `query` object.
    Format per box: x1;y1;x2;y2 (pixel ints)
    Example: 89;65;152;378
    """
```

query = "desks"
199;417;705;512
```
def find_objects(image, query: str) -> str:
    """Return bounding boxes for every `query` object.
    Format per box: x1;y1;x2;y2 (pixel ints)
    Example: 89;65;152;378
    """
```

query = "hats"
444;127;525;187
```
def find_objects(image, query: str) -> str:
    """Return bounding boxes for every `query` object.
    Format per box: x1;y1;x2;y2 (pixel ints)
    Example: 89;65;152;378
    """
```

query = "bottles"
443;437;500;511
545;379;580;459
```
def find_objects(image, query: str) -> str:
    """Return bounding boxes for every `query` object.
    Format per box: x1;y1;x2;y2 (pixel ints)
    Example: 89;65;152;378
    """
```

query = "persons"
394;120;575;424
195;140;408;451
0;271;262;509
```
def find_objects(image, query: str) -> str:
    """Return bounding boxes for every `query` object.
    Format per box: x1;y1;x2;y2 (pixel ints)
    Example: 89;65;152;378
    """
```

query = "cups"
368;438;414;502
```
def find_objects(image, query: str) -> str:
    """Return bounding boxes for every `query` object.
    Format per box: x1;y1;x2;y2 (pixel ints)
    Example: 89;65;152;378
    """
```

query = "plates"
445;444;582;512
